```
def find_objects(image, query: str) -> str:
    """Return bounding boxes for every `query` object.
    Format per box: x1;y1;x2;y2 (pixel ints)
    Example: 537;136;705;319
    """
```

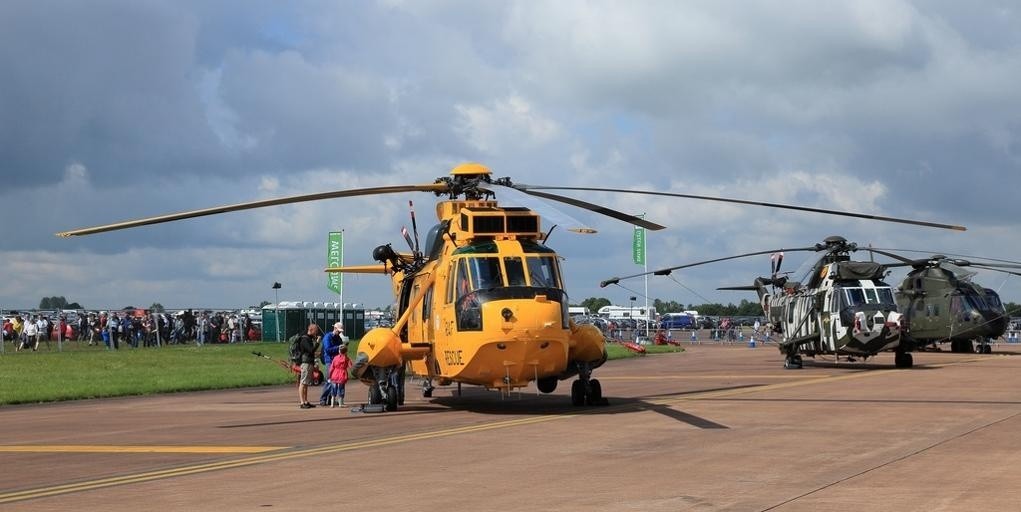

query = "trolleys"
251;348;324;387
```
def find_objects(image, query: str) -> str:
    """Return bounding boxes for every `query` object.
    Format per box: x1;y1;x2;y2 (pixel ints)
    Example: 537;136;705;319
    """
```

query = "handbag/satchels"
320;344;324;364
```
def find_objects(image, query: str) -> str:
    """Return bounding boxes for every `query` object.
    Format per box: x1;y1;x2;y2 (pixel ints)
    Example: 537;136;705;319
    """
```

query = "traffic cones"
690;331;696;341
1007;333;1013;344
618;329;622;341
1013;333;1019;344
738;332;744;340
749;335;755;348
634;335;640;346
666;331;672;339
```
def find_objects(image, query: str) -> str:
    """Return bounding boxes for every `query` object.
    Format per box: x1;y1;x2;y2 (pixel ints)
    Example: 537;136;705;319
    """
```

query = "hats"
334;322;344;332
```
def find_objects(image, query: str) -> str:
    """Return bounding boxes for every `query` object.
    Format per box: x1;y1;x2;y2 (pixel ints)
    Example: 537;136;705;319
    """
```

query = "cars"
0;308;262;335
571;303;768;332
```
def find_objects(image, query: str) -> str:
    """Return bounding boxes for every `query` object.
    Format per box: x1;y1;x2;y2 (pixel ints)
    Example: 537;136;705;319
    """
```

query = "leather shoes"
301;402;315;409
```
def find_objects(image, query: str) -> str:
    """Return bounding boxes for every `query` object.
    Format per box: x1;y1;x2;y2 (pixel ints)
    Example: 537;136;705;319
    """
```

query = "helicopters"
601;236;1020;370
864;247;1020;354
52;166;968;411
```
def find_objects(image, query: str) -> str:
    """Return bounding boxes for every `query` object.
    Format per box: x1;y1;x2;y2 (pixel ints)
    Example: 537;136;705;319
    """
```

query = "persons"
327;345;354;409
298;324;323;408
591;314;776;345
320;323;350;405
0;308;260;351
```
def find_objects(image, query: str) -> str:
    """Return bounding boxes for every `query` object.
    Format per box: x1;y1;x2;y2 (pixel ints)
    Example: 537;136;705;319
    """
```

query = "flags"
326;231;343;296
633;227;644;267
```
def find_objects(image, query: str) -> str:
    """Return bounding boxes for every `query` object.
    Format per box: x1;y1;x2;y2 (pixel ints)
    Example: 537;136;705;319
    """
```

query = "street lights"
629;295;637;332
271;282;283;342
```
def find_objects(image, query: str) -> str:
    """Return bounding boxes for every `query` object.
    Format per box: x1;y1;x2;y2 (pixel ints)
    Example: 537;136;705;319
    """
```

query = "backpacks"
289;334;311;365
